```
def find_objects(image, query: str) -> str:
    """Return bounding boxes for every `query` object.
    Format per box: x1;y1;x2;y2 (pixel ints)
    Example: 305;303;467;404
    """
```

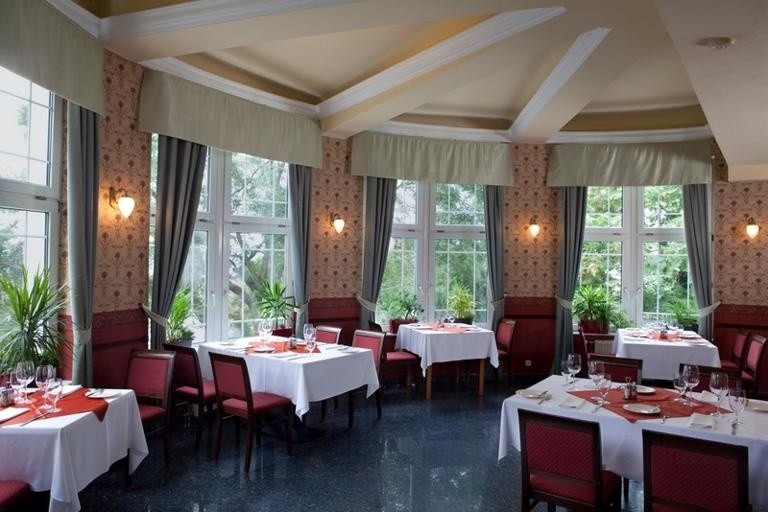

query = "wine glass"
36;366;55;409
302;324;317;359
560;361;574;389
728;388;746;425
259;319;274;351
10;360;35;405
673;372;687;402
439;308;456;327
46;379;63;413
709;372;728;417
589;361;612;405
568;352;582;385
683;364;701;407
638;314;684;343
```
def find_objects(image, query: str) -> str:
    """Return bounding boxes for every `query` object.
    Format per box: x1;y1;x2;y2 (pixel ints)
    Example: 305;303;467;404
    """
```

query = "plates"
298;340;320;345
635;385;656;393
272;337;289;341
516;389;550;399
273;352;300;357
626;328;701;339
623;404;661;414
461;325;475;329
85;390;118;398
24;388;37;393
415;326;432;329
750;400;767;411
338;349;359;353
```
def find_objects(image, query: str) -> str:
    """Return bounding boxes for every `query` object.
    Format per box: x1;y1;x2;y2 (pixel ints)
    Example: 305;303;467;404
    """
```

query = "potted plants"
256;277;297;337
674;300;698;333
163;283;194;343
0;257;80;388
446;281;477;325
380;290;425;334
572;284;628;333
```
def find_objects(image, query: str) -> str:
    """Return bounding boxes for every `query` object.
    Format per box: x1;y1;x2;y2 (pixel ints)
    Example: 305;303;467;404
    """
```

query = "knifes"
536;390;552;405
19;413;50;426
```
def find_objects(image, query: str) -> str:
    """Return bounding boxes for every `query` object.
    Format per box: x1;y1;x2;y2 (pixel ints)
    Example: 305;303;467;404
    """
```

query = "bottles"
632;382;637;398
624;377;632;400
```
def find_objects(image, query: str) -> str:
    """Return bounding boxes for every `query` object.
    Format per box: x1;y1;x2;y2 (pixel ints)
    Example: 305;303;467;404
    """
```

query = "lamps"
528;216;541;238
746;217;759;240
109;185;136;218
330;212;346;234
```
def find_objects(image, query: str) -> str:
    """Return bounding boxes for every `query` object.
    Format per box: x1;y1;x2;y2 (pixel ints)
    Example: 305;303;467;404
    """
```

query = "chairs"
0;477;28;512
127;349;178;464
